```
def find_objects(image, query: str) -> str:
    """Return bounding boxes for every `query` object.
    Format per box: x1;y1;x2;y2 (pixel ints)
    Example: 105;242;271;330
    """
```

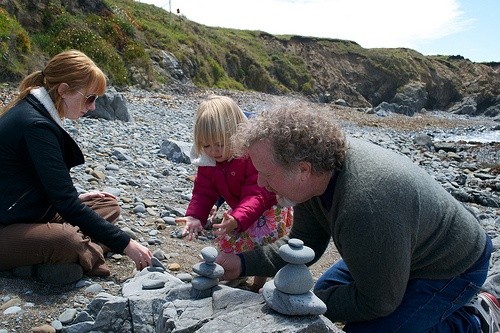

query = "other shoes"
10;259;85;284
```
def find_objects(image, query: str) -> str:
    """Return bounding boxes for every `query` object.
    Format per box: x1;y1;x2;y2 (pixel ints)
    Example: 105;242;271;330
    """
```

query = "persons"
174;95;293;255
213;98;500;332
0;50;155;286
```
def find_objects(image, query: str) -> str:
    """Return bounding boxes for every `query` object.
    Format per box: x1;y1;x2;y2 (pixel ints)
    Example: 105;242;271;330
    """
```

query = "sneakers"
462;291;500;333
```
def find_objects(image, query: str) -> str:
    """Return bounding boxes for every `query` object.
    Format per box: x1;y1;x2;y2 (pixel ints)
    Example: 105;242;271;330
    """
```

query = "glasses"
70;86;98;106
